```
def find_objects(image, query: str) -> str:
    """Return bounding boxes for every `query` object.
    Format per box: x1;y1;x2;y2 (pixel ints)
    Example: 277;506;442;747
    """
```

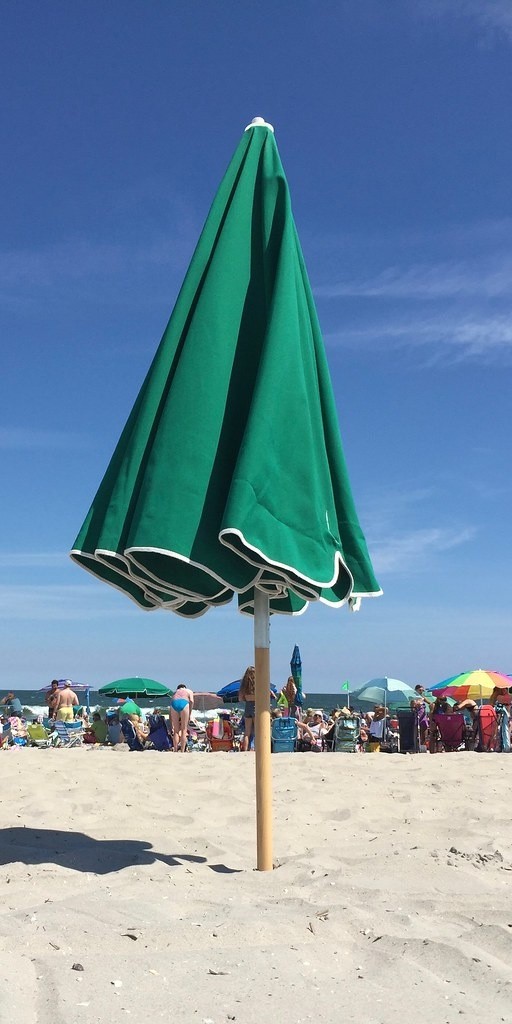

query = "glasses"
314;714;321;717
419;689;425;692
439;699;442;701
92;718;98;720
444;699;446;701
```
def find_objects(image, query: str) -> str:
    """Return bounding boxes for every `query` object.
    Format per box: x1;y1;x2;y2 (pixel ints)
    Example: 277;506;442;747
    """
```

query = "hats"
64;680;73;686
315;711;323;717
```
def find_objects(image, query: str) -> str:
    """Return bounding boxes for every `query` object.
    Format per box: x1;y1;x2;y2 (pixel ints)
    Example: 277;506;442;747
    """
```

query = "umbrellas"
99;676;174;704
38;679;93;690
216;679;277;703
425;668;512;704
290;642;304;707
193;693;222;721
68;117;383;870
351;676;422;741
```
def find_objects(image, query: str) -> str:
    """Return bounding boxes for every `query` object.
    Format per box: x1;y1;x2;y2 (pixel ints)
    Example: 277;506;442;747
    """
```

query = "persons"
272;709;317;745
492;687;512;713
239;666;255;751
83;713;107;742
1;692;22;719
370;705;388;738
411;685;435;732
302;708;326;737
130;715;148;745
45;680;61;718
454;698;478;718
433;694;447;712
170;684;194;752
153;707;162;720
53;680;79;723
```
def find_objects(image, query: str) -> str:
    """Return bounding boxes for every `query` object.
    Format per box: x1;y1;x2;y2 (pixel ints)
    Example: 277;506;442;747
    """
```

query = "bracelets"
310;737;314;739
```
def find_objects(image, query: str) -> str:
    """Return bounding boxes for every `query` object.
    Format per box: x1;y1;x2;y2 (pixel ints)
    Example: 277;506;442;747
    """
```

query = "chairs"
29;719;85;748
146;716;174;752
206;719;234;751
430;713;469;751
272;715;361;752
120;719;146;751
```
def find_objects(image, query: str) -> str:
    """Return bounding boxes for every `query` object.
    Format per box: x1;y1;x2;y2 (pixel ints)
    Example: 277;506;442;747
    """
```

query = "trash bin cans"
398;710;417;751
